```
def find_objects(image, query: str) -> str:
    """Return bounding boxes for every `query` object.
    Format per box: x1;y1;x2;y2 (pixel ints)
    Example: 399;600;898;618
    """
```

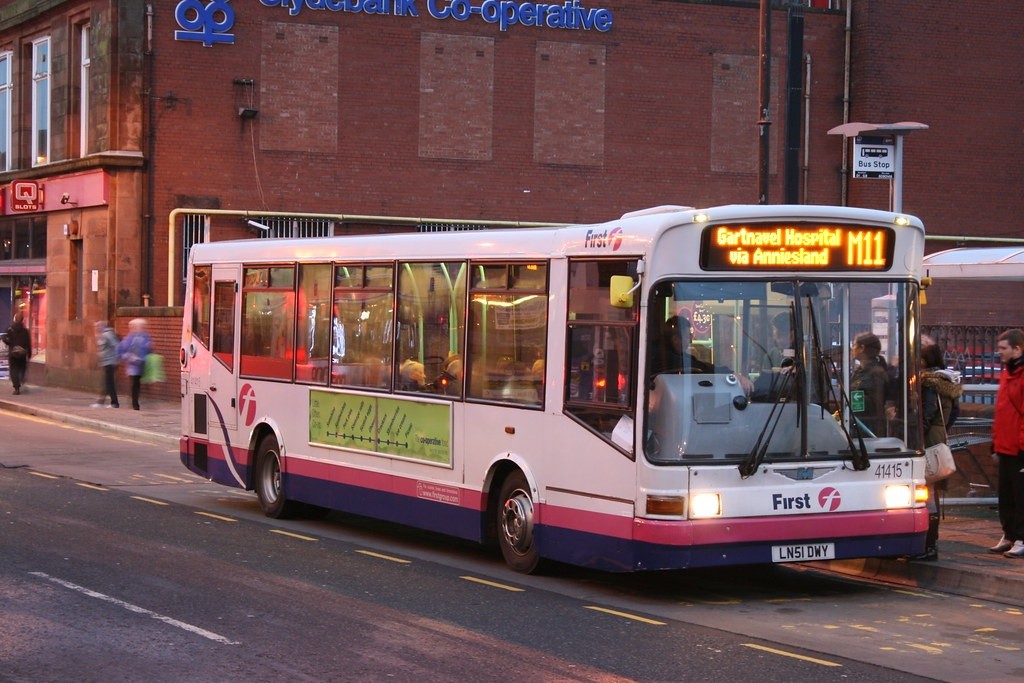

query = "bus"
180;204;932;572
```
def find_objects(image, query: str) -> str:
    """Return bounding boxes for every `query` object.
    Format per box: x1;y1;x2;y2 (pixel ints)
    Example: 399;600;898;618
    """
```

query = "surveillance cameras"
248;220;270;231
60;193;70;204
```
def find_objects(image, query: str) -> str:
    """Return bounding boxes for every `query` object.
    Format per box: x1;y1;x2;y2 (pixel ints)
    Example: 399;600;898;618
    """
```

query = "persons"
569;371;590;401
752;312;827;406
834;332;897;443
888;336;963;562
114;318;154;410
429;355;544;406
987;328;1024;557
397;358;434;394
90;321;122;409
646;317;755;396
2;313;32;396
363;358;388;387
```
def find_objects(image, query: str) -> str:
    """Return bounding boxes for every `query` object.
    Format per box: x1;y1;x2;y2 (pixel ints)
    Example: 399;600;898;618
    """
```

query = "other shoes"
106;405;116;409
911;549;938;562
1004;540;1024;559
90;403;103;408
12;389;20;395
990;534;1014;551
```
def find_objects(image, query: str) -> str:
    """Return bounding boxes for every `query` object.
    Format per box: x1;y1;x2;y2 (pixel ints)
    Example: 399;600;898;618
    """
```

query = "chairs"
347;350;544;406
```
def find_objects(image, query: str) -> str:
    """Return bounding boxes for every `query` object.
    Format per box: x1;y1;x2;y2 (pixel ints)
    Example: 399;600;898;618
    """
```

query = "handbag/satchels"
924;443;956;483
139;352;165;383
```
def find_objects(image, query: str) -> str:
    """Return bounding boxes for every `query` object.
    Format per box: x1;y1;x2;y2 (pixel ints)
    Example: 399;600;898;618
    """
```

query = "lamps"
239;107;260;119
60;192;78;205
